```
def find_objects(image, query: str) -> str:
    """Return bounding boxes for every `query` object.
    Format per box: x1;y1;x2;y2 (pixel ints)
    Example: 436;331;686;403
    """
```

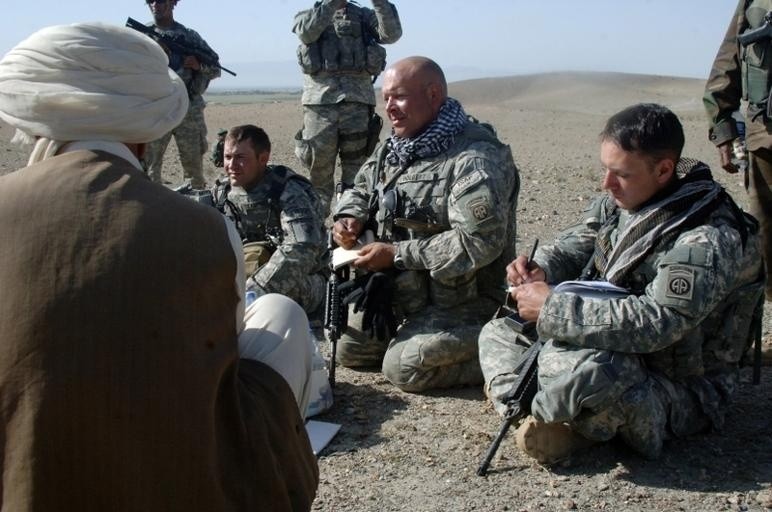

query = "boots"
515;414;573;466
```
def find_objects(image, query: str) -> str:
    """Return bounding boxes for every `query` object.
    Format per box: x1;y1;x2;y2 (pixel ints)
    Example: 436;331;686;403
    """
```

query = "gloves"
333;268;368;318
362;269;398;343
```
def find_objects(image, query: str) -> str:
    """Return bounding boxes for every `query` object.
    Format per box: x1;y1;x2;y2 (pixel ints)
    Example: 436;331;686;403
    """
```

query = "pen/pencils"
338;218;365;246
520;238;539;285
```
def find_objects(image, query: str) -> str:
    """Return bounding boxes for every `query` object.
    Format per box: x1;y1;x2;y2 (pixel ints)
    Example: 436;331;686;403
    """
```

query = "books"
501;267;633;304
327;228;375;273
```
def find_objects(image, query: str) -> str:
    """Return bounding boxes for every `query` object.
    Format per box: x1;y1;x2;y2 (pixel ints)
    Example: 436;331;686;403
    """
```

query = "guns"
735;10;772;129
366;108;384;159
123;15;239;81
324;179;377;394
474;249;599;479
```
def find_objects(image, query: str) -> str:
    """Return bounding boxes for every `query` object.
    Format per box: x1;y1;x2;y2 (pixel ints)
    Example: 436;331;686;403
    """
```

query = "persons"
134;1;223;200
700;0;772;310
475;100;768;467
204;122;321;327
322;54;520;393
0;17;319;510
290;2;404;226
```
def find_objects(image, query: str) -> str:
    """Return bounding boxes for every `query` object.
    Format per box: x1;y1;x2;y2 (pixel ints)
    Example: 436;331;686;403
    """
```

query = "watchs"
389;239;407;275
